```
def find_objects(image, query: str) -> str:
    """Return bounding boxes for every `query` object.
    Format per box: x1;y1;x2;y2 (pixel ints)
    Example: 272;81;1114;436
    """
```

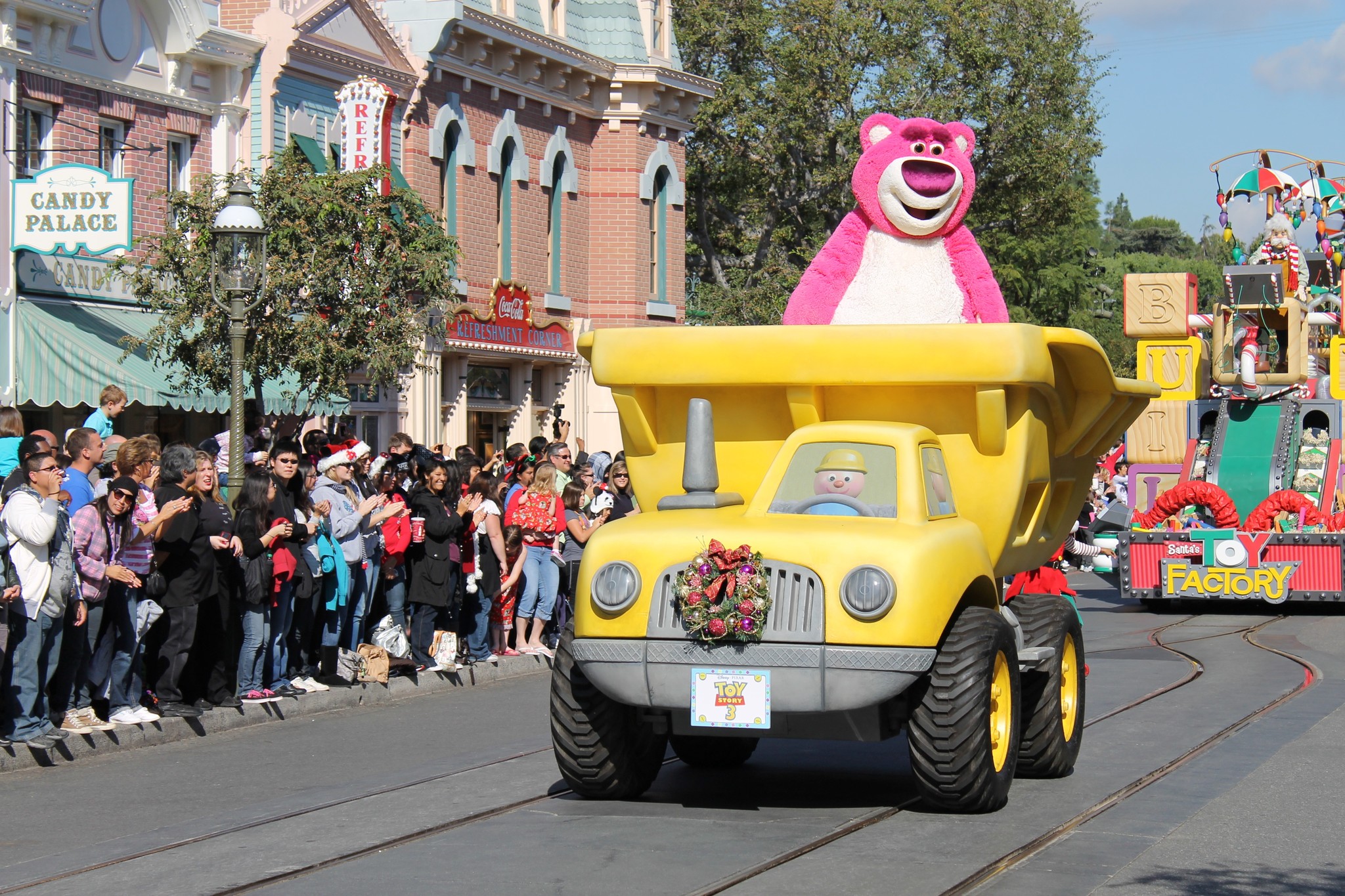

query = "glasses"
338;463;352;469
306;472;317;478
613;473;629;478
276;457;299;464
114;488;135;504
378;471;396;479
34;466;60;471
554;455;571;459
141;458;153;463
358;456;371;463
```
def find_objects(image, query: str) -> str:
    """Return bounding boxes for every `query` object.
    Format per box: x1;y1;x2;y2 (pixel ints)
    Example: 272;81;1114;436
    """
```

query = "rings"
173;505;177;509
319;505;322;507
462;499;465;501
458;500;460;503
291;527;293;531
475;500;477;502
466;502;468;504
240;552;241;553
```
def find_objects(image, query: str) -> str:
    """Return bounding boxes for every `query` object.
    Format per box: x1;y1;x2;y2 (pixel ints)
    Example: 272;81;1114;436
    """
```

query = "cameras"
53;469;66;478
552;404;570;436
438;445;444;451
220;531;231;544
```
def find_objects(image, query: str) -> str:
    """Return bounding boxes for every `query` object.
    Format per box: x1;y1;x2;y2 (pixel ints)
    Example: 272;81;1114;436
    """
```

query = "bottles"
267;554;273;561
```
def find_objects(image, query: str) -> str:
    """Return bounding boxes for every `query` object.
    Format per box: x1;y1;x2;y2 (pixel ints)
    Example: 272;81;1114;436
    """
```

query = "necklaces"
57;511;76;597
137;489;141;499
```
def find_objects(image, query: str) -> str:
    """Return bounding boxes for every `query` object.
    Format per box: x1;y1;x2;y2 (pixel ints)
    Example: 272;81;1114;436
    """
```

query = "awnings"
14;294;353;416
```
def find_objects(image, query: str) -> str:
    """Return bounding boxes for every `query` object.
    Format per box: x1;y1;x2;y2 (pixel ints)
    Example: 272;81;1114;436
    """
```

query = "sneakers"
213;696;243;707
289;676;316;693
44;726;70;741
157;703;205;718
49;708;93;734
133;707;161;722
260;688;283;702
304;676;330;691
20;732;56;749
108;708;143;725
237;689;271;704
184;695;213;710
77;706;116;731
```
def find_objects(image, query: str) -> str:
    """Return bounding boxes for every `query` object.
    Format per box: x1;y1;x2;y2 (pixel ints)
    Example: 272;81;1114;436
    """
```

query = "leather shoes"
0;735;13;746
273;685;297;697
287;684;307;695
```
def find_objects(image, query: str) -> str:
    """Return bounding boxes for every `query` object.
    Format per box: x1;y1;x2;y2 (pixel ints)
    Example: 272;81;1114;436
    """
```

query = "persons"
1004;439;1129;679
0;385;638;752
1247;213;1310;373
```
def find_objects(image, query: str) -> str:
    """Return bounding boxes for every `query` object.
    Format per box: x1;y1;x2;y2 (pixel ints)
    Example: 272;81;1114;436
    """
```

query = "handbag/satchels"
147;571;167;597
371;613;410;659
318;646;366;683
229;556;274;605
428;630;457;673
356;643;389;684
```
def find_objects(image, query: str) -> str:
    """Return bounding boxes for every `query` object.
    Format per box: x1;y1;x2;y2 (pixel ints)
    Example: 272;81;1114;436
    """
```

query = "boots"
315;643;352;687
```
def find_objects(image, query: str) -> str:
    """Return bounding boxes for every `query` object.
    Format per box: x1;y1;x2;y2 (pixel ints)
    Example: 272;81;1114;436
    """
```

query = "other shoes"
424;665;444;671
484;654;498;663
1079;565;1093;572
416;664;425;672
550;550;567;567
454;660;463;670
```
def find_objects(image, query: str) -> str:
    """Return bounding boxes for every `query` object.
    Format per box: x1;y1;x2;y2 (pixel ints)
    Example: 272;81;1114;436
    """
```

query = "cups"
411;517;425;543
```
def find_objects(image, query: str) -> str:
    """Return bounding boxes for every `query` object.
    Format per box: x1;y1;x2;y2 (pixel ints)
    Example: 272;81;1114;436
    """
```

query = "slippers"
518;647;555;660
490;646;520;656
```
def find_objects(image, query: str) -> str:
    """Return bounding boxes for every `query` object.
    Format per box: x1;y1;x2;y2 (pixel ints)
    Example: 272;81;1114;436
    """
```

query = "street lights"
208;170;272;523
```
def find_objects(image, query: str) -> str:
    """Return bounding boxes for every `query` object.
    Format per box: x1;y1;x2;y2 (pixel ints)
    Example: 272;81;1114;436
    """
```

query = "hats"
342;439;371;462
590;487;615;514
317;444;357;473
108;476;138;499
366;452;392;481
102;442;122;465
390;453;410;472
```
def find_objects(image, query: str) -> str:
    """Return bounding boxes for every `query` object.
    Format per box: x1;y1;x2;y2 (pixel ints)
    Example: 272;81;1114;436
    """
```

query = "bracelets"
149;520;157;530
266;533;275;537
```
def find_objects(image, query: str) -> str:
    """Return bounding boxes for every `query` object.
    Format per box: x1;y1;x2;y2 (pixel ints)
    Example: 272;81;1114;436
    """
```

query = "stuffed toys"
784;112;1007;324
